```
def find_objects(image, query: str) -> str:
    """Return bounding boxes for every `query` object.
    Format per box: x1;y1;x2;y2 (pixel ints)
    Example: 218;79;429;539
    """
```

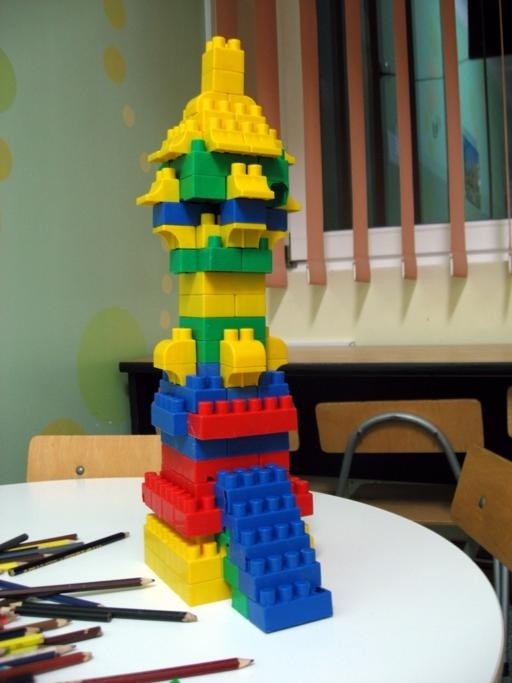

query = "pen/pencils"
0;531;255;683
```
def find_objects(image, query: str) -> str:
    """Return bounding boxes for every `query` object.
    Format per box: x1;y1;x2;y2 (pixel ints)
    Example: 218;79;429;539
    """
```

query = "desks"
1;476;504;683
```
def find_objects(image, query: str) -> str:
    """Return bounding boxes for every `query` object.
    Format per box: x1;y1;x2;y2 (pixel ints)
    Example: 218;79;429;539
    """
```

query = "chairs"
315;398;484;553
448;445;512;683
27;434;161;482
